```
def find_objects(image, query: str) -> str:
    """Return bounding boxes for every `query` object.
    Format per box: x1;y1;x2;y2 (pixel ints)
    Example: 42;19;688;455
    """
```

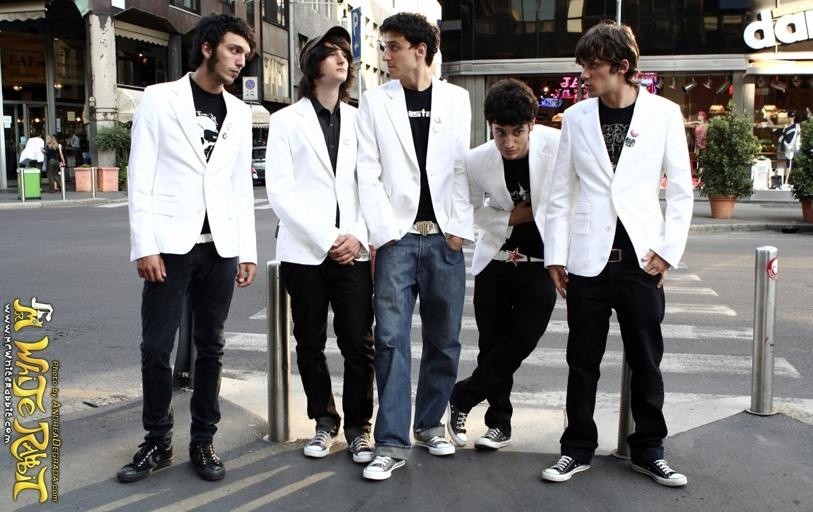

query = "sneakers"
349;432;375;462
543;455;591;482
448;398;468;446
633;458;687;486
415;436;456;455
475;427;512;450
118;440;174;483
188;439;225;482
304;429;341;459
363;455;405;481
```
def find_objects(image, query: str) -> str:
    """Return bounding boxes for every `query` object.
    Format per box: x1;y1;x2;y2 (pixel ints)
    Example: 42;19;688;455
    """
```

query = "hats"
299;26;351;75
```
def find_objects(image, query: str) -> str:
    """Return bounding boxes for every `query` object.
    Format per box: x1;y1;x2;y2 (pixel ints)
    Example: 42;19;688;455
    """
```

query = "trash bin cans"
18;168;41;200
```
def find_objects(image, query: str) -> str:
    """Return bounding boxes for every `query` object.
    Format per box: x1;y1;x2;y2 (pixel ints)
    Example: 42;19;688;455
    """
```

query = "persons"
261;23;378;464
540;21;693;489
692;110;712;191
66;130;80;148
442;79;563;451
761;107;801;188
355;11;474;482
18;128;46;193
114;11;256;484
39;134;64;194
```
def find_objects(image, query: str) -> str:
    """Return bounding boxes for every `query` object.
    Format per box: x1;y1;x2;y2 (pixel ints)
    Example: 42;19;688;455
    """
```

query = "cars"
251;144;267;185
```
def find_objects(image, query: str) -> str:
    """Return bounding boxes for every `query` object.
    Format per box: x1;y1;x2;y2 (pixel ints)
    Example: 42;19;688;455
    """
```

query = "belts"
409;220;440;237
608;249;636;265
494;248;545;265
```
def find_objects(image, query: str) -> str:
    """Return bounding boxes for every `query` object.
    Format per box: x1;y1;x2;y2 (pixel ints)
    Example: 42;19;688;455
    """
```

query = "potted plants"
693;99;761;219
787;115;813;223
